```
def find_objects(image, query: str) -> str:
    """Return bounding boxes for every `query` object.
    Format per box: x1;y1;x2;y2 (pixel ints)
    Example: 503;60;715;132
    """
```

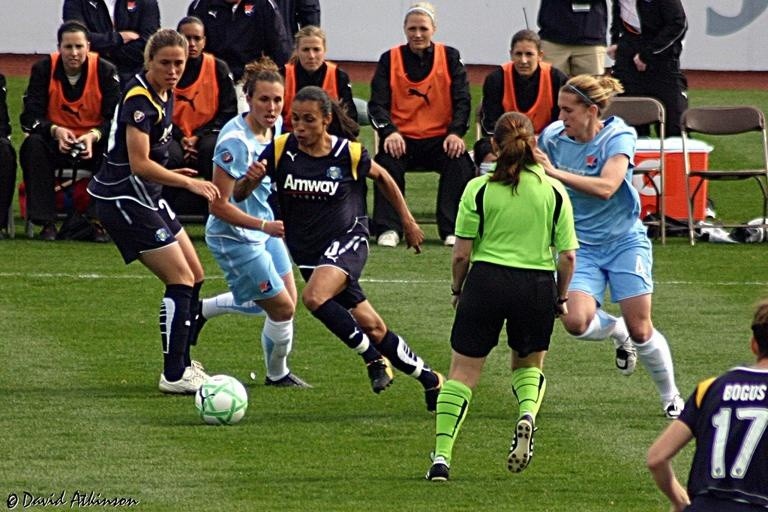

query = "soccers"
195;374;249;425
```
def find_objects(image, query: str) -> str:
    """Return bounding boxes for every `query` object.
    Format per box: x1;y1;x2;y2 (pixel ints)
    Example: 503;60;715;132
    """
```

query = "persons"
646;300;768;512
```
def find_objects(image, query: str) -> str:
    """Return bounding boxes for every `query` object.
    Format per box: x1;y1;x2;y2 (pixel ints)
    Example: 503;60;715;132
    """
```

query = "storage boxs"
628;137;713;226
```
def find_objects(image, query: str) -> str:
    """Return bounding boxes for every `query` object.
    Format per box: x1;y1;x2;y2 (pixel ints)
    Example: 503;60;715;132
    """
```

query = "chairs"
684;104;767;241
8;98;494;239
604;96;674;242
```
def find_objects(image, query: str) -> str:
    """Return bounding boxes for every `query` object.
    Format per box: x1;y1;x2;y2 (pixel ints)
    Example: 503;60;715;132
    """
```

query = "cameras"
70;141;86;159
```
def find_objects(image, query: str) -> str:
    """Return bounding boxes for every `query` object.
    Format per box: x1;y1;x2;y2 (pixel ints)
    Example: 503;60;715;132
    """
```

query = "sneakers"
665;398;684;418
40;225;58;240
367;355;393;393
378;230;399;247
508;415;534;473
425;371;444;410
615;317;636;375
160;360;210;392
426;452;449;481
265;372;311;386
191;300;207;345
444;235;456;245
